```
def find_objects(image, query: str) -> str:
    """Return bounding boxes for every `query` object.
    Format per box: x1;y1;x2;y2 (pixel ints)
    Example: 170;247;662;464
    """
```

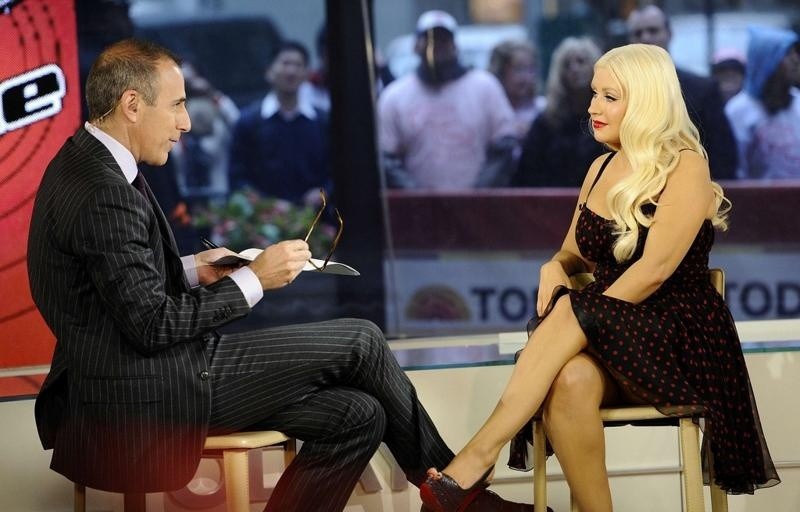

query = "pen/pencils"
200;237;218;248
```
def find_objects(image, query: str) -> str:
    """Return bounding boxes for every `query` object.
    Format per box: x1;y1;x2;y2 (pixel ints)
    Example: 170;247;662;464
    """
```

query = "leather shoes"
420;489;554;512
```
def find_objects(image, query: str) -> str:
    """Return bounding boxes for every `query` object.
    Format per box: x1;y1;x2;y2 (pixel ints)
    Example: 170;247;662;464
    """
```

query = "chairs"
533;267;728;512
74;429;296;512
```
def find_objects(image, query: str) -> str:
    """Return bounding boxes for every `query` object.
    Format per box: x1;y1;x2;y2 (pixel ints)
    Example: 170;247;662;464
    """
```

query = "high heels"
420;463;496;512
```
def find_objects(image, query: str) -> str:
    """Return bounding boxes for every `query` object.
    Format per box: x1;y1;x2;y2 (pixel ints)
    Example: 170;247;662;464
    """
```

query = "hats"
416;11;458;38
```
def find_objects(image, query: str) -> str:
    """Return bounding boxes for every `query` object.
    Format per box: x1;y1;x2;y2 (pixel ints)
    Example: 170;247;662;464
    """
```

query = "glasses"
303;188;344;273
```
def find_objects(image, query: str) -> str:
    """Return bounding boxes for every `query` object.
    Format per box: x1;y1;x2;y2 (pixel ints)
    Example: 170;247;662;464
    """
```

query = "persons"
623;4;741;181
486;39;549;159
228;39;340;208
26;35;555;512
512;34;611;186
707;46;747;100
377;10;524;191
167;63;243;199
722;23;799;182
419;43;722;512
